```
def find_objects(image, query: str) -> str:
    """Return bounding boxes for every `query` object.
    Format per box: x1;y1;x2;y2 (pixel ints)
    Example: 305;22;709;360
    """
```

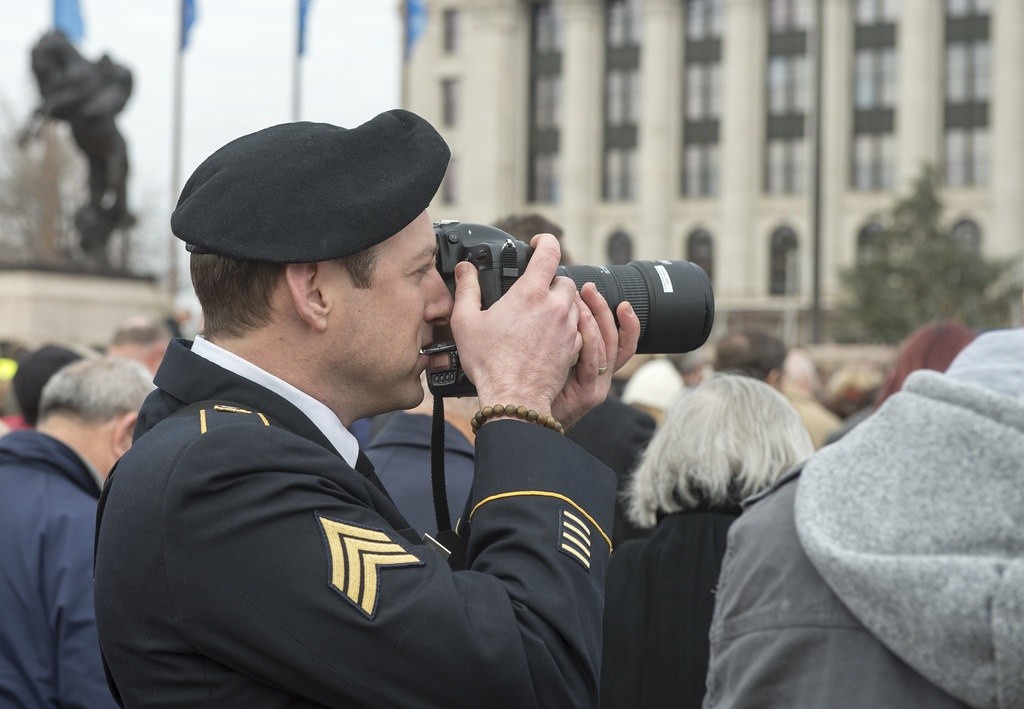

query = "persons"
705;330;1024;709
565;320;971;709
93;111;640;708
1;211;576;708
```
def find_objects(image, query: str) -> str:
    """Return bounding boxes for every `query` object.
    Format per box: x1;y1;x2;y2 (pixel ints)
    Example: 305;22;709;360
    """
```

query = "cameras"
424;219;715;397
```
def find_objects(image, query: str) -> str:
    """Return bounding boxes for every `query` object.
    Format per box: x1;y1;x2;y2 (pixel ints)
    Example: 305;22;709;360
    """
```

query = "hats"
621;358;684;409
169;108;451;262
11;346;85;424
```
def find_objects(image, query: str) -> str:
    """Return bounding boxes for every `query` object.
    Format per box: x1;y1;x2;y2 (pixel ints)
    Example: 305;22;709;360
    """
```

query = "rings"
598;368;607;374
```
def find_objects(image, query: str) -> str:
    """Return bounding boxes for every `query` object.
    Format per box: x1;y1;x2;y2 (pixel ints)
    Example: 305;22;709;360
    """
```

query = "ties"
355;448;398;507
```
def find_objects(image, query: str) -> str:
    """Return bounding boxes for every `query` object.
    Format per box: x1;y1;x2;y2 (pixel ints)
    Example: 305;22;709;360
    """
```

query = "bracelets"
471;404;565;440
